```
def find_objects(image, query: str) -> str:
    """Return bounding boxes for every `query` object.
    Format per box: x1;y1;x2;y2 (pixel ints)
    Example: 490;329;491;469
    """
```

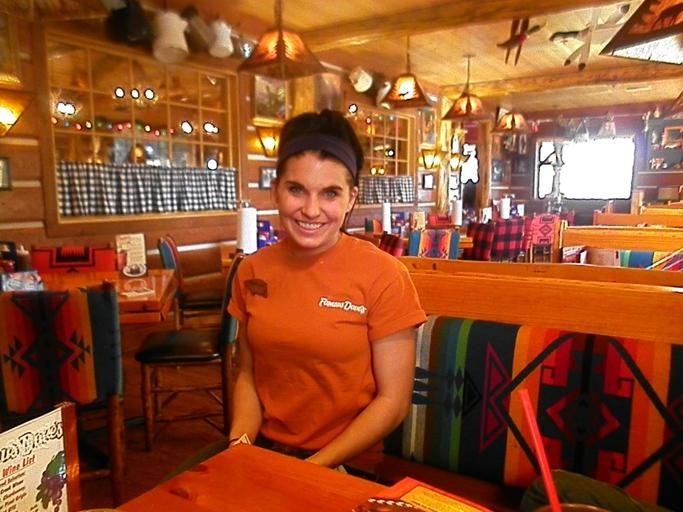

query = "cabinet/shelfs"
344;99;411;175
30;14;244;237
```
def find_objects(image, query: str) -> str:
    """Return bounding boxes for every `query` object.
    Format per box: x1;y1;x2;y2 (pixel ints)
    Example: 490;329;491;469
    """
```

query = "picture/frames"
251;74;290;125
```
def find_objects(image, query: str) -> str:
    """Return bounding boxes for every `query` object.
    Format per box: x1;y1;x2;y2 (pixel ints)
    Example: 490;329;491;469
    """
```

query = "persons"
162;108;428;482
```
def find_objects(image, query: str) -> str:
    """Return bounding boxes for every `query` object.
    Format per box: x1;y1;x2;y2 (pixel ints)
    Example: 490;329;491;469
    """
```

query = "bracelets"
228;438;240;447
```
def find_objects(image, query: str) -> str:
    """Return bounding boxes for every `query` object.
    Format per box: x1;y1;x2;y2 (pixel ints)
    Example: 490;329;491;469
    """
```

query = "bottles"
500;195;510;219
451;196;463;225
383;197;392;234
236;201;258;255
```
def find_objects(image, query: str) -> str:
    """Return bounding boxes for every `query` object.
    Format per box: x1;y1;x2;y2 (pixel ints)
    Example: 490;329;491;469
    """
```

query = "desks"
112;439;419;512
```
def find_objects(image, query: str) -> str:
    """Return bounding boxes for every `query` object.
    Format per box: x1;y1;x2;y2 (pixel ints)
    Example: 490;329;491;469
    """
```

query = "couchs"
147;238;224;307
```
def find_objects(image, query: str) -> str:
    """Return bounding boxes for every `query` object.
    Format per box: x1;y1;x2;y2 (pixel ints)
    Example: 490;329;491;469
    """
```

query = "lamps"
255;126;285;159
421;147;471;173
493;93;538;133
234;1;330;81
380;30;438;108
598;1;682;67
104;0;236;63
0;69;41;140
441;54;494;120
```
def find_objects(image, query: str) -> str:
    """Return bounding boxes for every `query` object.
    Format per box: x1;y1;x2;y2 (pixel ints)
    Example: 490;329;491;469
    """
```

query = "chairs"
134;251;245;452
357;208;600;267
0;280;129;512
156;232;183;331
0;241;19;276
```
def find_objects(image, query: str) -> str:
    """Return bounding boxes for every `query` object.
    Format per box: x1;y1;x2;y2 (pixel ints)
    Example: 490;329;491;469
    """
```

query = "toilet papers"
381;203;392;235
500;198;510;219
451;200;462;225
236;207;258;254
